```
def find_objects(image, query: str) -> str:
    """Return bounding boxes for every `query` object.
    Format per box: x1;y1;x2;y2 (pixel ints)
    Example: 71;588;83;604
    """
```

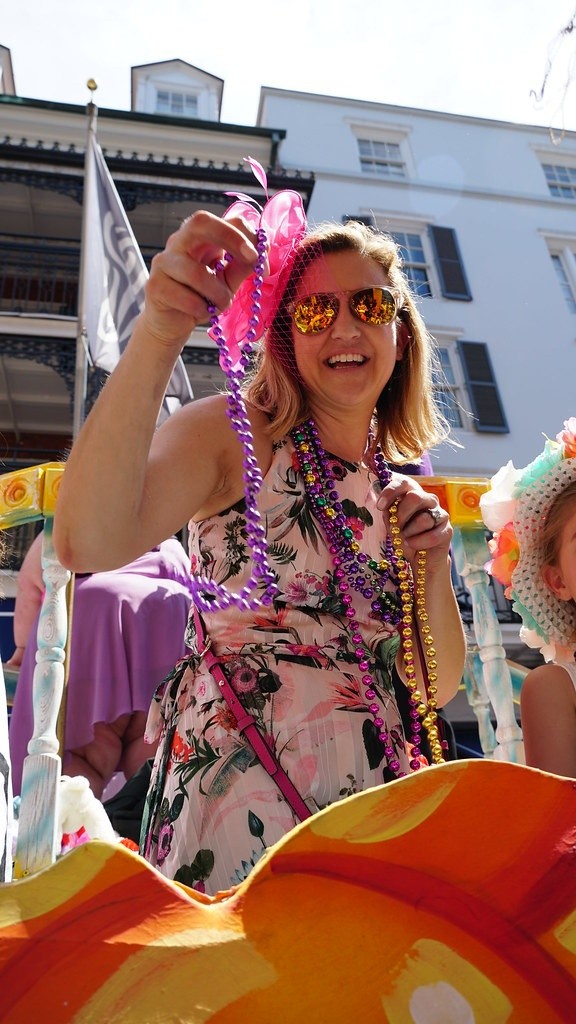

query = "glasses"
279;285;408;337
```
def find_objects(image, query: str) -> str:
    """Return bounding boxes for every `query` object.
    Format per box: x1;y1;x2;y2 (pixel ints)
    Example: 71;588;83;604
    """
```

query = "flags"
81;136;194;431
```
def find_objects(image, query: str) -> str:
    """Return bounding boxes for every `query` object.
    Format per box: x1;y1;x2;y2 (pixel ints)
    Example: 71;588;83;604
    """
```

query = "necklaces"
282;410;446;778
156;227;280;614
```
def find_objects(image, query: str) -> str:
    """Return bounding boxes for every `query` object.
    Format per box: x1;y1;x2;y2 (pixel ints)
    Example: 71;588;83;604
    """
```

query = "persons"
7;526;190;796
478;413;576;778
54;154;466;896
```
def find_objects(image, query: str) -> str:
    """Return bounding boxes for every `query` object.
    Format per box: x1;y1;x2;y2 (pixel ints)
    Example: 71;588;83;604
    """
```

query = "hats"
479;417;575;665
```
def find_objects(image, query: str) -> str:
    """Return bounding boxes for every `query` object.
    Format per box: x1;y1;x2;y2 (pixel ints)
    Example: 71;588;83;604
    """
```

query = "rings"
428;508;441;527
